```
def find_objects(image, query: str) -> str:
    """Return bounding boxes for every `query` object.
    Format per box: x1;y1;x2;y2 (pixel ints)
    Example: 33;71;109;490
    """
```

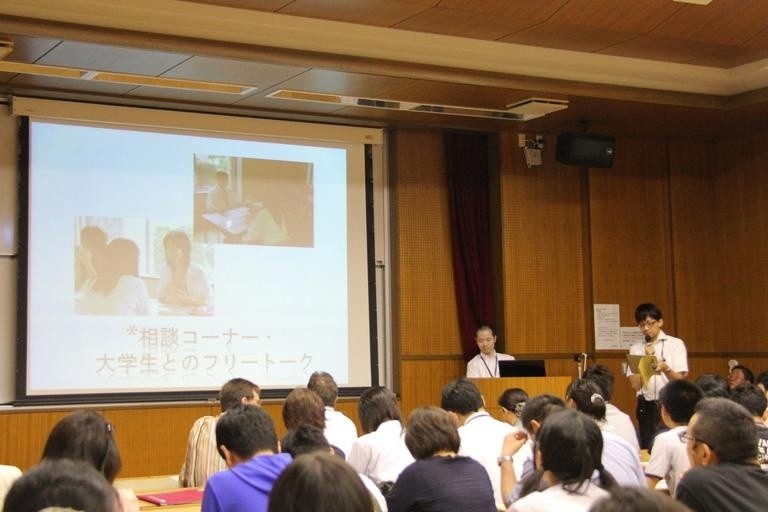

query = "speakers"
555;132;616;169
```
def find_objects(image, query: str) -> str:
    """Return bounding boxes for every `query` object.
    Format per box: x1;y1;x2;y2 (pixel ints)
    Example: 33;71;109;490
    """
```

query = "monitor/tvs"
497;359;546;377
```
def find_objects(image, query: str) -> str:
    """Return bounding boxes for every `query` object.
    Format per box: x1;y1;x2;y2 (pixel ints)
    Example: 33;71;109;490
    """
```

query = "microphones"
643;334;652;344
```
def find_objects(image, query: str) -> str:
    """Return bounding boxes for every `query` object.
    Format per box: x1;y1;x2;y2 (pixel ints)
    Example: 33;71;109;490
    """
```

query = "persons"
206;170;236;215
77;228;210;317
38;410;139;512
3;459;123;512
627;305;690;451
467;325;515;378
244;189;289;244
178;365;768;511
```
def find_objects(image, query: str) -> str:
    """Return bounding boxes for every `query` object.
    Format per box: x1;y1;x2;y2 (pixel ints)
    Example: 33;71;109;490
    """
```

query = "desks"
137;484;207;512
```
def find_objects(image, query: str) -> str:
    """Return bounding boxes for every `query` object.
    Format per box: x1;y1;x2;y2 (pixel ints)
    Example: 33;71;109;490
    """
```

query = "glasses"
677;428;714;452
636;319;660;328
